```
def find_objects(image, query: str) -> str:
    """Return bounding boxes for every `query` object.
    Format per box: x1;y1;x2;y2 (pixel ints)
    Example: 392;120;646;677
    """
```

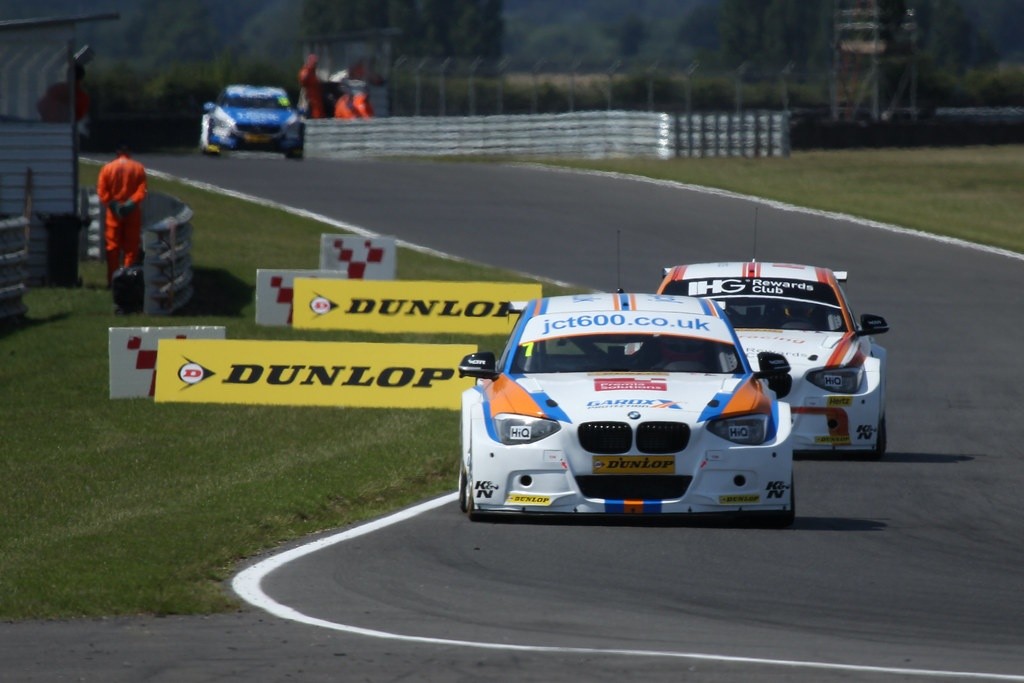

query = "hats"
309;55;318;63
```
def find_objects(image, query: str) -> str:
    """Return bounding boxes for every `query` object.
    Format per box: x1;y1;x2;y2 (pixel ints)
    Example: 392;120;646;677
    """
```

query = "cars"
657;259;887;459
456;291;795;526
200;85;305;161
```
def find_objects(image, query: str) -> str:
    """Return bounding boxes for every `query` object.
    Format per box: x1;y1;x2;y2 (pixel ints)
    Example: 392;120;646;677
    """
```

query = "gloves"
120;200;136;217
109;201;121;217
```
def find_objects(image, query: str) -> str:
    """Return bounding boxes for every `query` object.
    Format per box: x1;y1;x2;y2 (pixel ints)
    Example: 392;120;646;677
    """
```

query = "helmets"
660;338;706;362
784;294;815;319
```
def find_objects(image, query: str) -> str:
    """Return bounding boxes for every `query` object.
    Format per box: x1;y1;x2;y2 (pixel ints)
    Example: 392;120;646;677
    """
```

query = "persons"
785;302;813;320
335;92;372;118
97;144;147;289
657;338;705;364
297;54;327;118
